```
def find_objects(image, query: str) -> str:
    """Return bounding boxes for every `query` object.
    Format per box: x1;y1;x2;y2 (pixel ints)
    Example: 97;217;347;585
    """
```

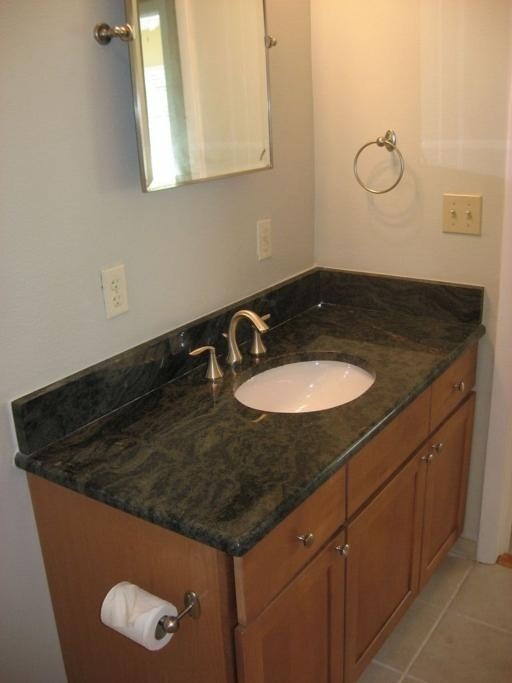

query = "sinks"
231;349;377;417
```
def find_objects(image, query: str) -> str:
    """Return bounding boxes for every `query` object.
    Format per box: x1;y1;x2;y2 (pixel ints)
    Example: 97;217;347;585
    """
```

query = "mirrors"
93;0;275;195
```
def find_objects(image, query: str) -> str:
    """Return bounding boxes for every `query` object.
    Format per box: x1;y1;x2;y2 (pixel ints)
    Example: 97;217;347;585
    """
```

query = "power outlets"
254;219;275;262
101;264;132;317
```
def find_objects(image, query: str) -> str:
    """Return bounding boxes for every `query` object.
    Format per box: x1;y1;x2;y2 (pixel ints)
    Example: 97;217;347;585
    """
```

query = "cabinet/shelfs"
24;341;483;681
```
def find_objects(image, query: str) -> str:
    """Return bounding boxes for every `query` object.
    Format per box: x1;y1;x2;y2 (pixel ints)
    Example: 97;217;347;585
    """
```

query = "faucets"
225;304;272;367
